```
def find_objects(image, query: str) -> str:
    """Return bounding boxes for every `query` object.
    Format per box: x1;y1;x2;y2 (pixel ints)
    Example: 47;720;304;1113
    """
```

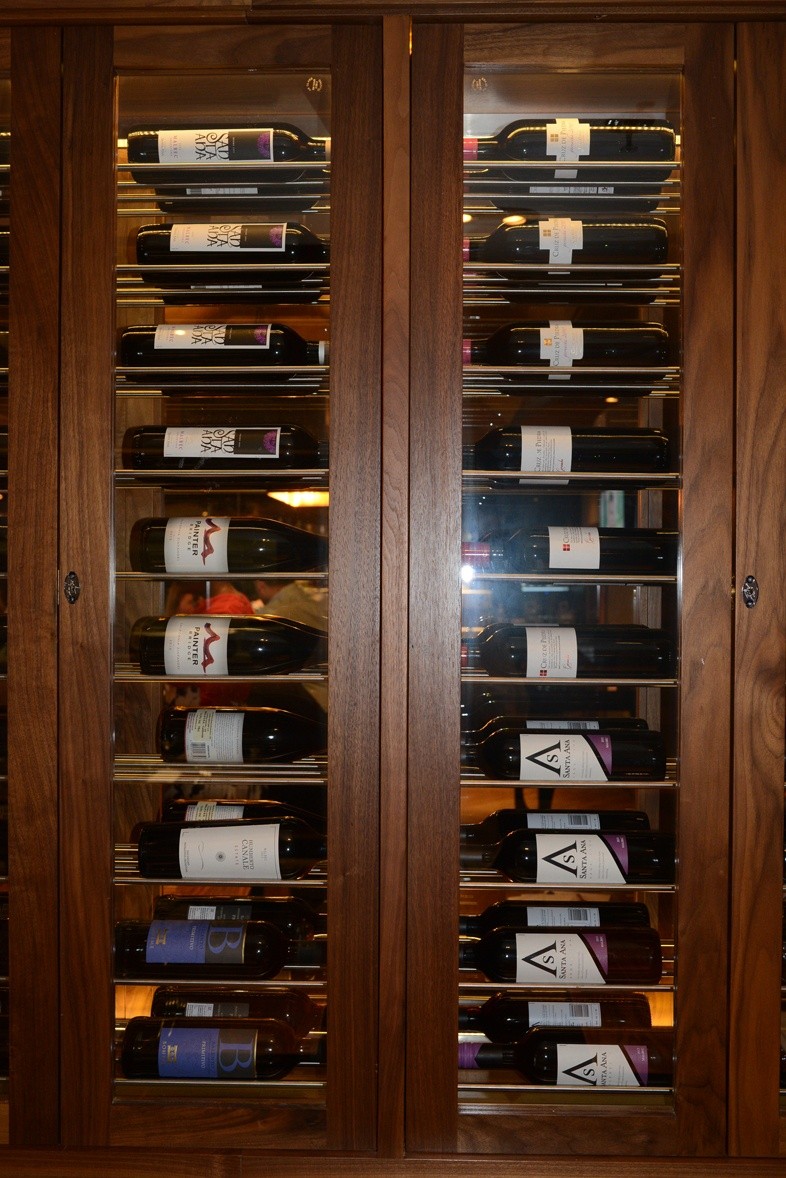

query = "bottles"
463;117;676;1087
111;124;333;1078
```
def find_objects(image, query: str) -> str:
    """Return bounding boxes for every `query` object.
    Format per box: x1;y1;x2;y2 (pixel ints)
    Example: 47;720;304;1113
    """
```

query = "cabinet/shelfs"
0;0;786;1178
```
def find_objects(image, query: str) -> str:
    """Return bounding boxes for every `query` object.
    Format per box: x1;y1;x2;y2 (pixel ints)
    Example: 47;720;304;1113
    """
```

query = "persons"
178;580;257;615
255;576;331;724
